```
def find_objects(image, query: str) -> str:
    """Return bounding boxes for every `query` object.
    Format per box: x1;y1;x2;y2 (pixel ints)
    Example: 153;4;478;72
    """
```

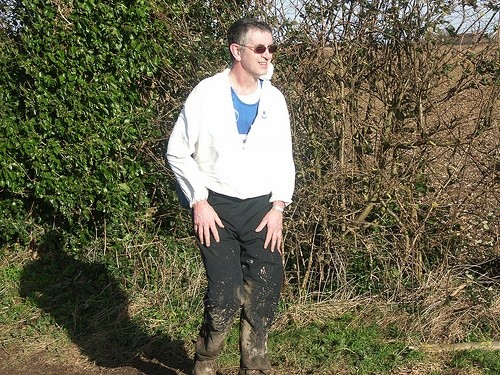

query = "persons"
166;19;294;374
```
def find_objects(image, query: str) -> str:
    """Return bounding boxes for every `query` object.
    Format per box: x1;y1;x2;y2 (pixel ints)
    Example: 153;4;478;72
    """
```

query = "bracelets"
273;205;284;212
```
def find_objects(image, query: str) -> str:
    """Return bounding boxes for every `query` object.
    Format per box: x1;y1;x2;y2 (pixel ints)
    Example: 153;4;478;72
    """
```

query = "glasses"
239;44;277;53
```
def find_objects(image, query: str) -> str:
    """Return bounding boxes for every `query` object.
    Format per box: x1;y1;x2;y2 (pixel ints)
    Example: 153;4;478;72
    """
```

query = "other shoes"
192;361;217;375
238;368;266;375
